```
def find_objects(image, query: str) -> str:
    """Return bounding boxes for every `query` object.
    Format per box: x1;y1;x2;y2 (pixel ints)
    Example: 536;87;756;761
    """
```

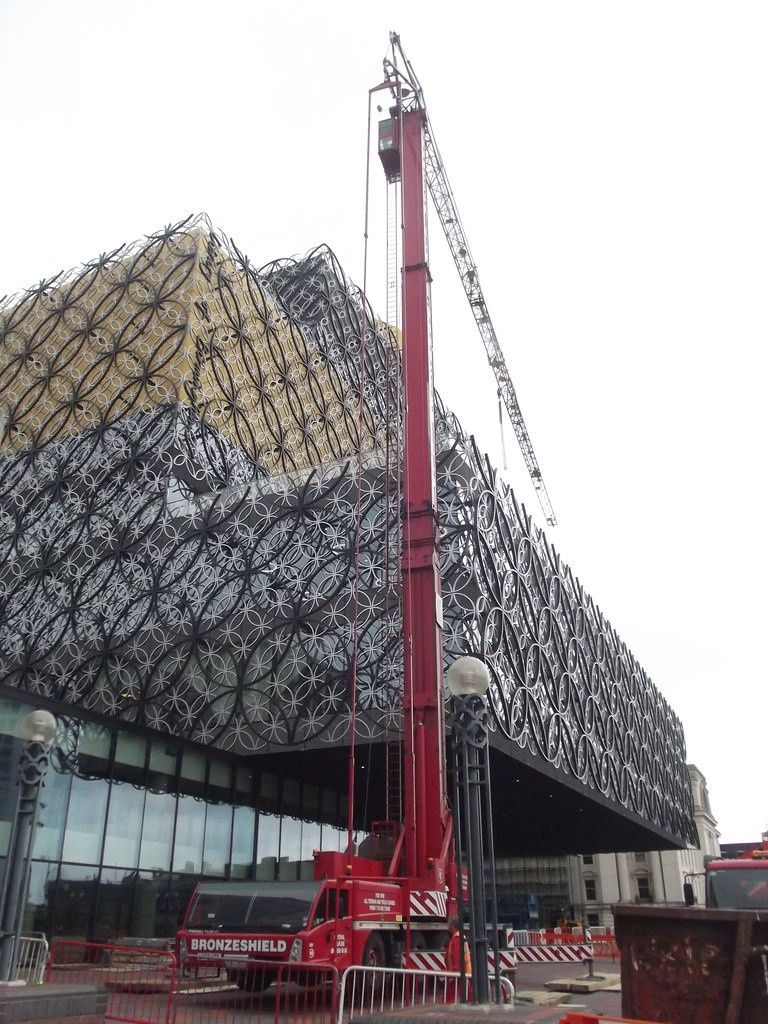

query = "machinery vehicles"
173;32;606;1006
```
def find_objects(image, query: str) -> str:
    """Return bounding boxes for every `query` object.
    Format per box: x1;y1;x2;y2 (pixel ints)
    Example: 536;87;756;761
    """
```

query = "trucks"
684;850;768;912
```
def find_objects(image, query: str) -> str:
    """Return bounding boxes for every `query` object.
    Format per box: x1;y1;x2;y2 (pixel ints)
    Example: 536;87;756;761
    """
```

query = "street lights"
0;710;58;981
445;655;501;1006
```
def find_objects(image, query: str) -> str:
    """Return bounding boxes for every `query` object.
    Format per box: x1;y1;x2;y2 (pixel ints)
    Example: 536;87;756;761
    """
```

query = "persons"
445;915;471;1003
